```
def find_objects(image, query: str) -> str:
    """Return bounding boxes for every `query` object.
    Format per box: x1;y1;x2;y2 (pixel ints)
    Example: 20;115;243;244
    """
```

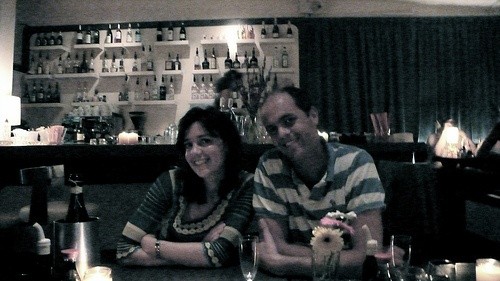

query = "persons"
251;86;405;275
214;69;250;115
117;107;254;265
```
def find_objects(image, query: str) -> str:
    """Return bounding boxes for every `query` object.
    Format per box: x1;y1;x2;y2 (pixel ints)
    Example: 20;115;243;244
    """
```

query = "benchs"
0;179;259;258
361;139;500;248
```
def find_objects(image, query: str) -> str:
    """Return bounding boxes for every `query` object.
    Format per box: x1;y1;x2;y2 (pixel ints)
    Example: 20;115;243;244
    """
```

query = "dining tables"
95;256;428;281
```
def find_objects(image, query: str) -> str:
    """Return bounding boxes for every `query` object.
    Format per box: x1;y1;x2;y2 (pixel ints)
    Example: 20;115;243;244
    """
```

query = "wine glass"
390;234;411;281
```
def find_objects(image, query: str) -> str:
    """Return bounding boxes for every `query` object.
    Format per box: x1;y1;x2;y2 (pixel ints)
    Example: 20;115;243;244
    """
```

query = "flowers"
310;209;358;281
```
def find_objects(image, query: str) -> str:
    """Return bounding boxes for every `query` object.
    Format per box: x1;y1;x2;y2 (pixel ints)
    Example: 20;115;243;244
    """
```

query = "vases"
310;247;339;281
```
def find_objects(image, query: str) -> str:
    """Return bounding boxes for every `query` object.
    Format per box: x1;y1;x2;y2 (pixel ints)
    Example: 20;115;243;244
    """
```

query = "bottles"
23;16;293;103
33;222;53;281
18;168;54;281
63;172;90;223
361;239;378;281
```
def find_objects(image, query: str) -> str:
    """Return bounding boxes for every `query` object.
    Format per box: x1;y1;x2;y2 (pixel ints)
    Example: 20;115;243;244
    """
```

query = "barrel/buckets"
53;216;101;281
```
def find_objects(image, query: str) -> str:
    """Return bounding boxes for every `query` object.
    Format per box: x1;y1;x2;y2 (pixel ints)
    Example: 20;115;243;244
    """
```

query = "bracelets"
155;239;160;259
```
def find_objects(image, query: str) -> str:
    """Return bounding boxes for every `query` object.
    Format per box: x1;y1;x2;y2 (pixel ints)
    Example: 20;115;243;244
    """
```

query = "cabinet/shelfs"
20;22;300;142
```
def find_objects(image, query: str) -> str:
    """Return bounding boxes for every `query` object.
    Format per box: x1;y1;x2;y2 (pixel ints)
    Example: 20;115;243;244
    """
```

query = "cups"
12;125;67;145
427;259;454;281
238;234;259;281
